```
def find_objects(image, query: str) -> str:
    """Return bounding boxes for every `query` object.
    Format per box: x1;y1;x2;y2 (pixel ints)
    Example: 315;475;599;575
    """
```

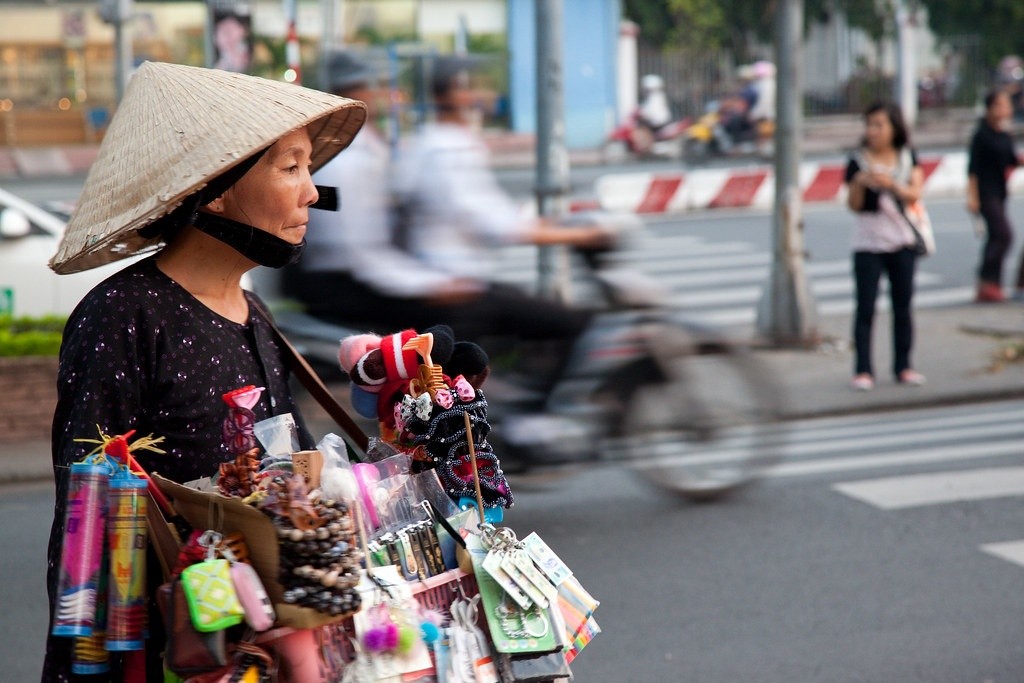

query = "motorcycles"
278;226;783;503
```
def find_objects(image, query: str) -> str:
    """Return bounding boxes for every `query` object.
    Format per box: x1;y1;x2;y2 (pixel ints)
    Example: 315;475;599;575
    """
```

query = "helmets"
735;60;756;81
641;75;666;89
754;62;778;78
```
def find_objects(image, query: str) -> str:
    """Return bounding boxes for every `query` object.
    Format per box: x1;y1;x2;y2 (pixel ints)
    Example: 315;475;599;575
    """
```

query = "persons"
845;101;937;389
633;57;785;157
968;92;1021;301
287;48;486;377
393;54;619;448
37;93;332;679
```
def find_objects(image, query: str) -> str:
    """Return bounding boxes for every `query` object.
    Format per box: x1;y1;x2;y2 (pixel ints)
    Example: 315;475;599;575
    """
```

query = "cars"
0;181;165;321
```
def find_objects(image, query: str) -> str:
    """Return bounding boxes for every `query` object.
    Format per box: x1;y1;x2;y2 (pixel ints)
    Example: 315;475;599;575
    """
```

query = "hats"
49;60;368;277
319;43;412;91
434;53;501;96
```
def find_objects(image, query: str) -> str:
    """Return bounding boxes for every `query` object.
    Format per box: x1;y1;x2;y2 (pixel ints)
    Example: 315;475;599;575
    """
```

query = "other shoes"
500;405;602;459
977;278;1010;301
850;370;877;391
896;368;927;387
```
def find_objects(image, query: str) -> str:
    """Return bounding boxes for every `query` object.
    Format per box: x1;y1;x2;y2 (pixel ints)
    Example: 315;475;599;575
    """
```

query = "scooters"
601;113;694;165
685;102;775;165
